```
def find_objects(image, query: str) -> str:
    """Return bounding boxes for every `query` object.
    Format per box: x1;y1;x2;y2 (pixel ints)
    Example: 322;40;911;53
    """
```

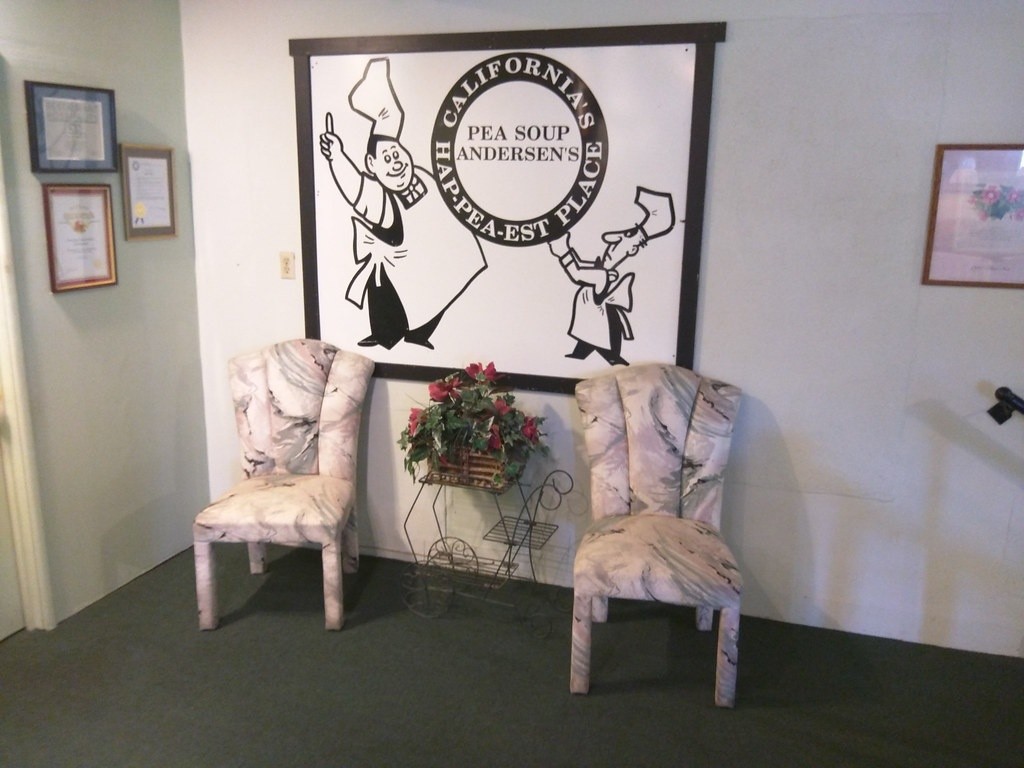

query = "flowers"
397;362;551;486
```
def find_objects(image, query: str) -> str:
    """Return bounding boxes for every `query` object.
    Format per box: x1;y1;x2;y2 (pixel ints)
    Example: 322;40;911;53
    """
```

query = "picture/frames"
922;145;1024;289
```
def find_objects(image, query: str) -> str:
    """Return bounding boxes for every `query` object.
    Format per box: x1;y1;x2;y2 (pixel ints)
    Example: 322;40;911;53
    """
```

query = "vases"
442;443;529;487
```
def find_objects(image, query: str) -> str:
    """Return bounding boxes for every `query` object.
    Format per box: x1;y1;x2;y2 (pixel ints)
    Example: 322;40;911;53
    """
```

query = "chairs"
193;339;375;630
570;364;744;707
